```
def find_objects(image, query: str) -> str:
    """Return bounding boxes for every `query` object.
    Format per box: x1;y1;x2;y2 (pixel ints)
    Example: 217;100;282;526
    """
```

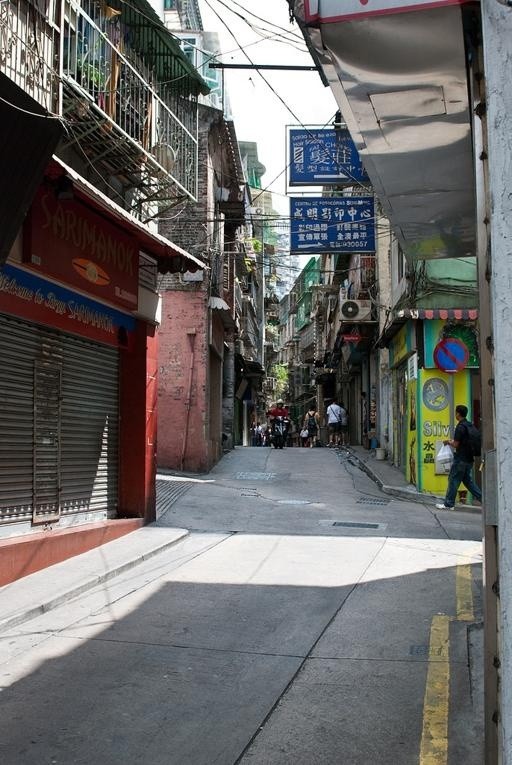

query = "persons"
360;392;370;435
251;399;349;449
436;403;483;510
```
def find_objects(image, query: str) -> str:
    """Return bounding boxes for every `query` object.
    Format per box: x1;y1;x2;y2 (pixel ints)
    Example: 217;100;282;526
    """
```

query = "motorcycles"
269;415;291;450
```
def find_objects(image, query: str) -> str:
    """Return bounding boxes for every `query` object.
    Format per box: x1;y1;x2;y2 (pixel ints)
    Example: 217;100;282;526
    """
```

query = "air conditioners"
339;299;371;321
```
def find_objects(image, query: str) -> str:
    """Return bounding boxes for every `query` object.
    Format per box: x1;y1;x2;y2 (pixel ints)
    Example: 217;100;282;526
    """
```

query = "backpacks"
460;423;481;456
308;412;316;426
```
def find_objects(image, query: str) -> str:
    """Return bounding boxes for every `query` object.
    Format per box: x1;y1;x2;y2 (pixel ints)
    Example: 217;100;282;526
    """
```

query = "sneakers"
436;504;454;510
329;441;345;448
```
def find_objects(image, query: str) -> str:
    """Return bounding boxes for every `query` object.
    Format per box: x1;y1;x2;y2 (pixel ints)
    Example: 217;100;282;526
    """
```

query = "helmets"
277;399;283;404
